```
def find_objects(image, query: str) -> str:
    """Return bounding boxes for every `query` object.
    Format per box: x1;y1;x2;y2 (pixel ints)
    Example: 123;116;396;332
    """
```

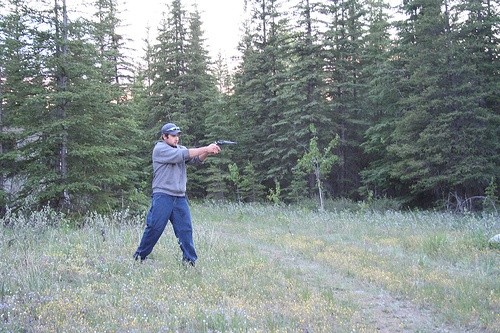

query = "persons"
132;123;221;268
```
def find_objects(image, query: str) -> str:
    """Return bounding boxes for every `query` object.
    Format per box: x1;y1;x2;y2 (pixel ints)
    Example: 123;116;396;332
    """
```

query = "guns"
214;140;238;146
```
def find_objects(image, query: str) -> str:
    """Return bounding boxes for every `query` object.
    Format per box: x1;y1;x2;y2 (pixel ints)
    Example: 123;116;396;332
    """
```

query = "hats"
162;123;182;135
